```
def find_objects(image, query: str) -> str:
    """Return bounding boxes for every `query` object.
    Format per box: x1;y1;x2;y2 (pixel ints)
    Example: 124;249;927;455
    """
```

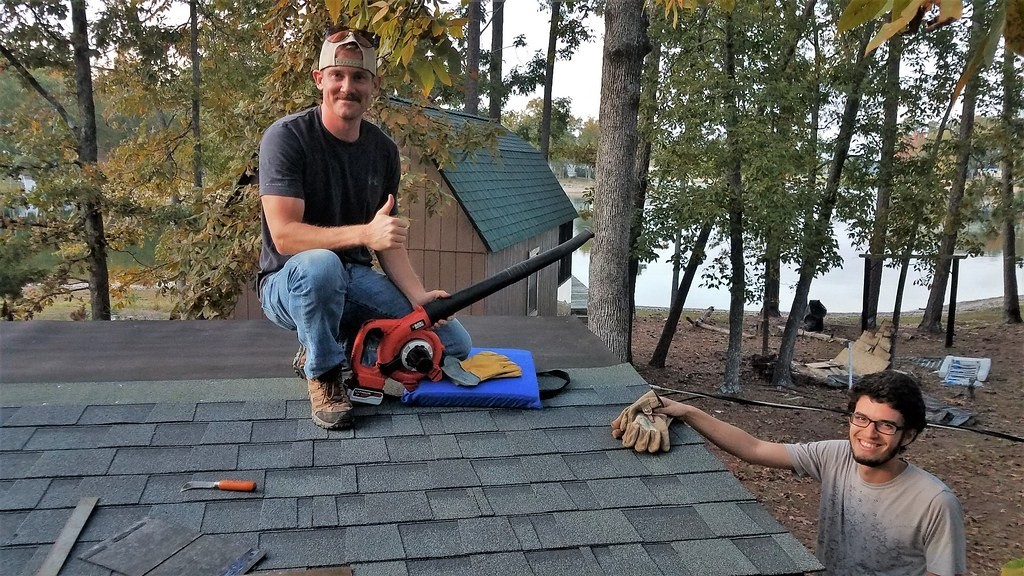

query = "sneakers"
293;344;309;378
306;366;355;430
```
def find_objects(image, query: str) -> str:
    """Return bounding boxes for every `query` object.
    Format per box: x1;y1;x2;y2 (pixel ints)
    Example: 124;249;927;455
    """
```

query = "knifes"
180;480;257;492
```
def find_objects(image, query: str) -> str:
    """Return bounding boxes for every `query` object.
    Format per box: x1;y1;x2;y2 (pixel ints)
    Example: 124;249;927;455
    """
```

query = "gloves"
610;390;676;454
442;350;523;387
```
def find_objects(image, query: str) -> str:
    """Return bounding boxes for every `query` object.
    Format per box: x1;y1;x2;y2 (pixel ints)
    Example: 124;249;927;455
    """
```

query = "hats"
320;30;377;77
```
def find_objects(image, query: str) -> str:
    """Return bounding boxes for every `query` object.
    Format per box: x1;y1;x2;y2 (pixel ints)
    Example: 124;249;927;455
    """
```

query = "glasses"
848;411;909;435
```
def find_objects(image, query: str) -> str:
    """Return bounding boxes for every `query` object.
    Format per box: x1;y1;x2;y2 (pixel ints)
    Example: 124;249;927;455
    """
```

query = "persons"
653;371;968;576
256;30;473;429
